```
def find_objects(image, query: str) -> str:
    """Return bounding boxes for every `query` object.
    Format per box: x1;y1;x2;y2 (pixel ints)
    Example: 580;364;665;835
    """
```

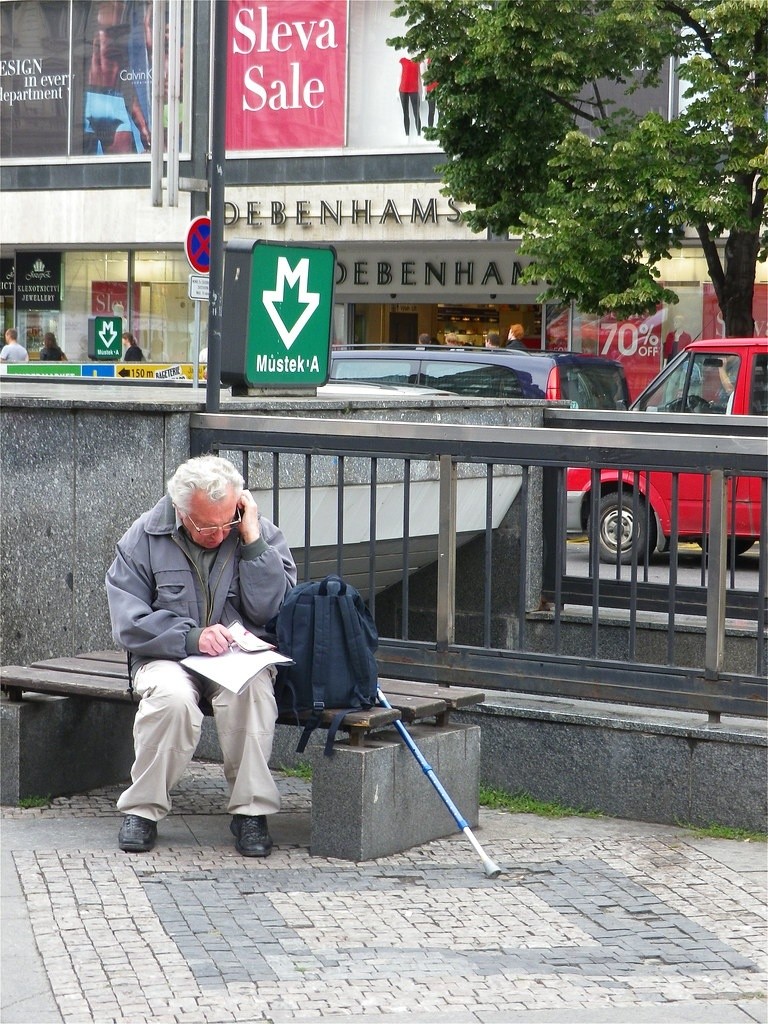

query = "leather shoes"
230;812;272;857
117;812;157;850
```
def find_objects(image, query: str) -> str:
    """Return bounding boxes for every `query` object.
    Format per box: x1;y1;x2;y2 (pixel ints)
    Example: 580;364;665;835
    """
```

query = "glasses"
184;504;242;536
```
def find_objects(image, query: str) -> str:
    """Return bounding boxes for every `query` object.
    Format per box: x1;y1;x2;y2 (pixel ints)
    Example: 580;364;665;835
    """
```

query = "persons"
104;455;297;858
718;358;741;415
485;333;500;349
39;331;68;361
416;332;433;352
445;332;465;353
505;322;530;350
80;1;183;156
661;314;691;368
0;327;30;363
398;55;440;138
120;333;150;363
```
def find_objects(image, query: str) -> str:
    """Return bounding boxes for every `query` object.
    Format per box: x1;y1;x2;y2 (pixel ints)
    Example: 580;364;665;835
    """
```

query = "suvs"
328;344;631;411
564;337;768;565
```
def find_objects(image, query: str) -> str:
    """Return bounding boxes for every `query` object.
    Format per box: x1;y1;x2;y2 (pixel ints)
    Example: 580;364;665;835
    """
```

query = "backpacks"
267;576;379;758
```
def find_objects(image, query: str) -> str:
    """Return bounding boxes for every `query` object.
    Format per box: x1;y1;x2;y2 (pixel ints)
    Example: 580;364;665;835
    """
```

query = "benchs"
0;649;485;860
